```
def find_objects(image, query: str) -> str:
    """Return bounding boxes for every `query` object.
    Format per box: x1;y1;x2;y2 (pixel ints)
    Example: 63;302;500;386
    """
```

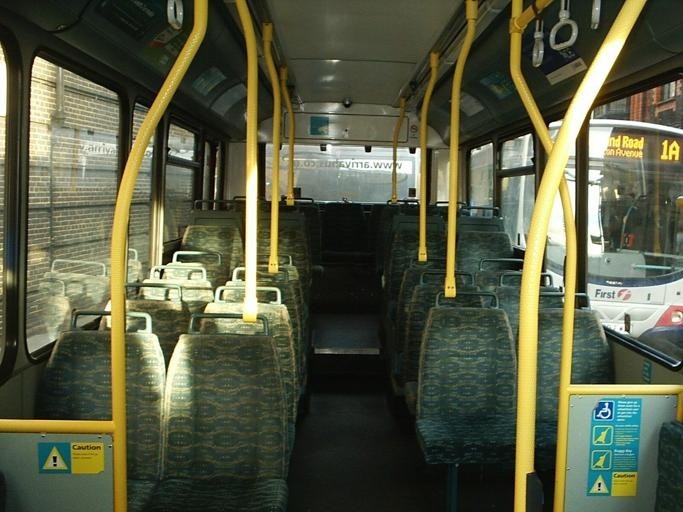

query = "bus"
264;146;422;203
468;118;682;340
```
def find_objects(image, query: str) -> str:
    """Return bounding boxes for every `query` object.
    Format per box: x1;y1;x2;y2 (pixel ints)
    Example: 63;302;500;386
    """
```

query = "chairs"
36;310;166;512
146;313;290;512
34;248;144;343
321;203;370;264
